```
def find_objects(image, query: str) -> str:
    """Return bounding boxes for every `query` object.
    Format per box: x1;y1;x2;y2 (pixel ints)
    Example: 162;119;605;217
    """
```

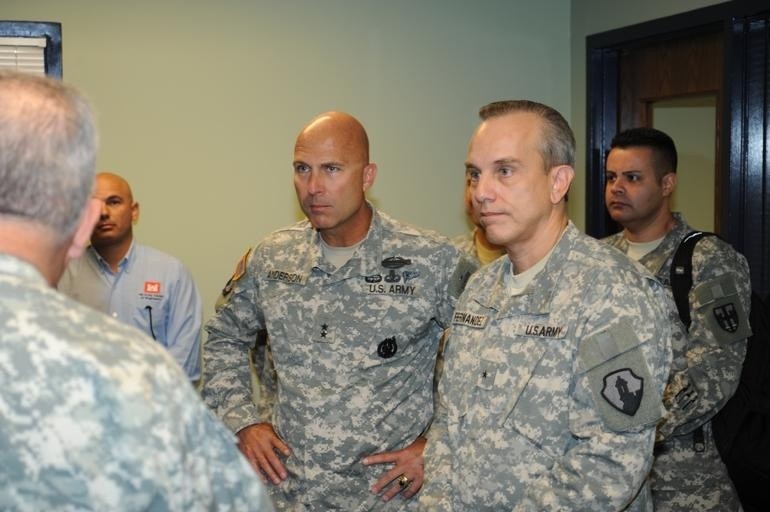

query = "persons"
0;69;279;512
54;172;203;395
201;110;477;512
396;97;674;511
437;134;509;266
593;125;757;512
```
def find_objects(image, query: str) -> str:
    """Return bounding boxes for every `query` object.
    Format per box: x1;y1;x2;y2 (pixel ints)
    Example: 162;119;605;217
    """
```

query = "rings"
398;476;408;487
